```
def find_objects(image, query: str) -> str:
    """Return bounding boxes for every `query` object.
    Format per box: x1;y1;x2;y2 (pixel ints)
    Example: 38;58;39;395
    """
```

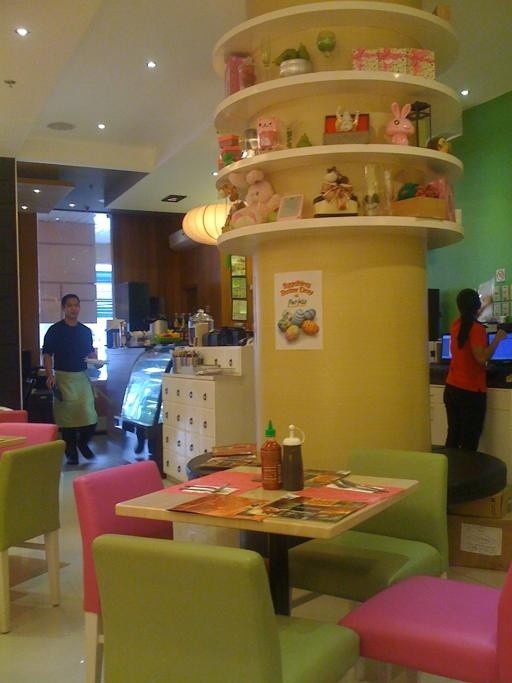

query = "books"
198;456;257;468
211;443;254;457
251;468;352;487
167;491;274;521
236;491;367;524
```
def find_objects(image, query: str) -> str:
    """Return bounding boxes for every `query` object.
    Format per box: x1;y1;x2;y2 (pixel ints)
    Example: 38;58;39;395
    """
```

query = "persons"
443;289;507;452
43;294;104;464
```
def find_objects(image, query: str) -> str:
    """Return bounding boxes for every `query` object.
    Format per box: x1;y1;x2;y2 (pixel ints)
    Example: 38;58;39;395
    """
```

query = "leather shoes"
79;445;96;460
67;457;80;467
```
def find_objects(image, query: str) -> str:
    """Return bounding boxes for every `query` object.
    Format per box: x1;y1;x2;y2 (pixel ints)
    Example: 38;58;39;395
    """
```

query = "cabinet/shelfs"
114;346;173;453
161;346;254;483
211;2;466;451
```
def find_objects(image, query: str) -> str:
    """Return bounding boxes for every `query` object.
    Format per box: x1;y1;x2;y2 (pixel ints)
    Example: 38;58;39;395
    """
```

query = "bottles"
260;420;279;491
281;423;305;492
172;310;195;333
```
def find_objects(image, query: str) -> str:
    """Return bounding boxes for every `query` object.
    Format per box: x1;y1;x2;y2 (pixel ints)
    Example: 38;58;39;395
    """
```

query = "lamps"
182;196;230;246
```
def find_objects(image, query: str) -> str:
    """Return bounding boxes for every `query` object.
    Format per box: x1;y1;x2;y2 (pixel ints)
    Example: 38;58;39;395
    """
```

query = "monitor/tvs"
441;335;452;359
487;332;512;360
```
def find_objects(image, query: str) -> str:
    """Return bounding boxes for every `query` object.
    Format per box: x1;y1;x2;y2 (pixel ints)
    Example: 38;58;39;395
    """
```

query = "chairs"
0;424;59;448
92;533;360;683
337;567;510;681
0;411;26;423
1;440;66;634
73;460;174;682
265;446;444;682
